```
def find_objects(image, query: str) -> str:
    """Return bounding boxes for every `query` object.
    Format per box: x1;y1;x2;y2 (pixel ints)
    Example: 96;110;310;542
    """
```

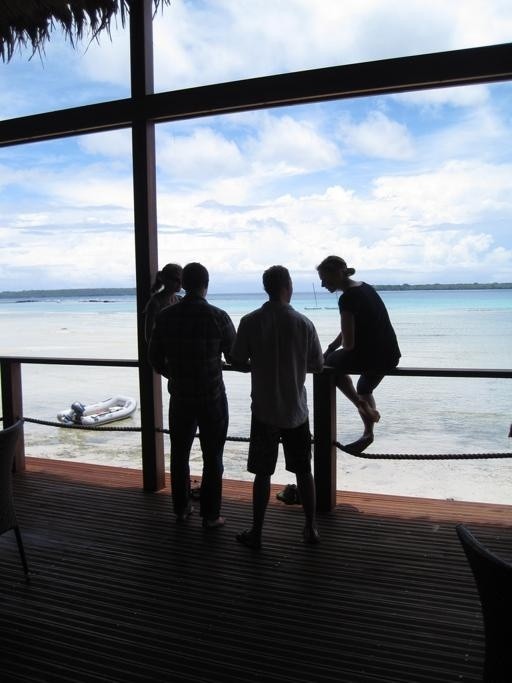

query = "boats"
56;395;136;427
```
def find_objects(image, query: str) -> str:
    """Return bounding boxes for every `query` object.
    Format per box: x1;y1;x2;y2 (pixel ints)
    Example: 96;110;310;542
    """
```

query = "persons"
317;254;400;453
229;264;325;550
143;262;184;343
147;261;237;527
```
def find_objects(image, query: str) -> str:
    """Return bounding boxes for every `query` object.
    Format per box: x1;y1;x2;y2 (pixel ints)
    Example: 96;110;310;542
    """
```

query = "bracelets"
328;343;336;352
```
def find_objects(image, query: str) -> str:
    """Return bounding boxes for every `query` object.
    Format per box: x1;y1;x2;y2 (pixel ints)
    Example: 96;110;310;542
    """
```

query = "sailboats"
304;283;322;309
324;288;340;310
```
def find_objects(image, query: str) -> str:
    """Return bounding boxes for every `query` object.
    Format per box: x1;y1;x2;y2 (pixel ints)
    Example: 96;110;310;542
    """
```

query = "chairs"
0;417;39;576
454;523;510;681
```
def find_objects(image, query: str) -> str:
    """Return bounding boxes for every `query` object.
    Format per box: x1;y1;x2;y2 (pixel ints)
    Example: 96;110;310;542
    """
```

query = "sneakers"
203;516;226;529
175;504;193;521
276;484;302;504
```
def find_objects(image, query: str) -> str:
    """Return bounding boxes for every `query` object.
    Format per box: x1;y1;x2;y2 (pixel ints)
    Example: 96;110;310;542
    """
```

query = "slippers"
302;526;321;544
235;529;261;548
